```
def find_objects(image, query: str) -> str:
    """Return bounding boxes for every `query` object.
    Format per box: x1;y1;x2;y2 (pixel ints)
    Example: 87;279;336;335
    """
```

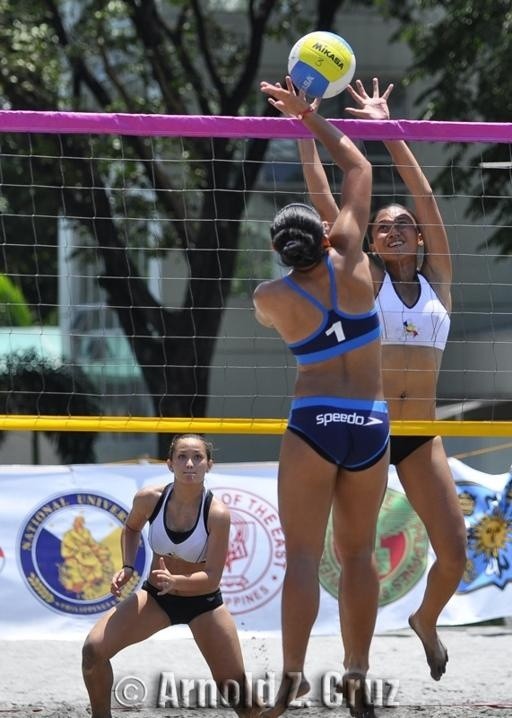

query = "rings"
165;580;170;588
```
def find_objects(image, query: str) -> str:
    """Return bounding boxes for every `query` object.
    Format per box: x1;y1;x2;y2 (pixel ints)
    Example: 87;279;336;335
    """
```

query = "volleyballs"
288;31;356;99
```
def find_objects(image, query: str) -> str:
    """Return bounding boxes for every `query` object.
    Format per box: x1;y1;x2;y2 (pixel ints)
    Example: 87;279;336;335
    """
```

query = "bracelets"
298;109;313;119
121;563;137;573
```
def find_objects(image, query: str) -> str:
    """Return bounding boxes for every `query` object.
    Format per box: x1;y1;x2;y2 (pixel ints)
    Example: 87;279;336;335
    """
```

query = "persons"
252;69;394;718
265;72;464;693
82;433;262;718
